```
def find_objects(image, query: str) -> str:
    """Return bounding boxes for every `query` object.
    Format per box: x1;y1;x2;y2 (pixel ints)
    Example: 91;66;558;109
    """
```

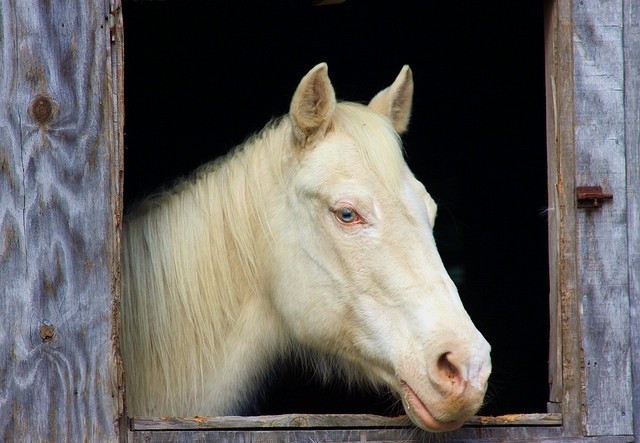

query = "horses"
121;62;493;443
